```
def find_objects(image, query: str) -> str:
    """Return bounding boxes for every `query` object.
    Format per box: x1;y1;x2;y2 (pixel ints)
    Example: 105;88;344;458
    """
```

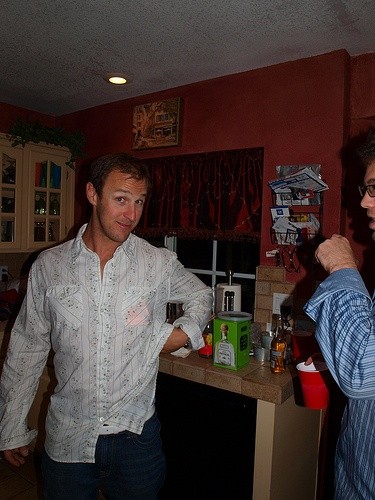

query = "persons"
0;156;214;500
300;125;375;500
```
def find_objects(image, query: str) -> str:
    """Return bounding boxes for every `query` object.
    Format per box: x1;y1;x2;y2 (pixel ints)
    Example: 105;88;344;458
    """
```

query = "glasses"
358;185;375;198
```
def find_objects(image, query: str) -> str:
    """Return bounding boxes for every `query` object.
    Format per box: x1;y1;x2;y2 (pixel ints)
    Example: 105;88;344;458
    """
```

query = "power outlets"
272;293;294;315
0;265;8;281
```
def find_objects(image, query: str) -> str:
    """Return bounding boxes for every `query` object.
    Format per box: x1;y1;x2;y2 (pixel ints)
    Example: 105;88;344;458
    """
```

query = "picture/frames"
131;96;181;150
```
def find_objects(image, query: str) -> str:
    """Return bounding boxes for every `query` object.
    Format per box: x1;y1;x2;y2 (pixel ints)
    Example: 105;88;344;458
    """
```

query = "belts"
98;404;156;437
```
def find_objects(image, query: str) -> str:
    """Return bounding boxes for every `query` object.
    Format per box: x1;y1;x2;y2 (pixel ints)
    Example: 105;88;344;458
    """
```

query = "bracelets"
179;325;190;349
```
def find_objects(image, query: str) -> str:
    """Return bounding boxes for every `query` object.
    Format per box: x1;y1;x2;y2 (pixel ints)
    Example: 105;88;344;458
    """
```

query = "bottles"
34;221;56;241
215;323;235;367
35;193;60;216
270;314;287;374
197;310;212;359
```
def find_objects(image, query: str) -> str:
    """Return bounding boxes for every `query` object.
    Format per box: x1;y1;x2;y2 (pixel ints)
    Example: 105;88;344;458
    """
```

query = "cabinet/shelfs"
0;132;76;254
267;162;330;274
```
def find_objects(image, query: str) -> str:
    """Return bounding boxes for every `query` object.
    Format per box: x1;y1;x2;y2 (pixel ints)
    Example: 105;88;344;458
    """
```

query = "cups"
254;345;270;361
290;330;313;365
296;361;332;410
249;322;261;355
